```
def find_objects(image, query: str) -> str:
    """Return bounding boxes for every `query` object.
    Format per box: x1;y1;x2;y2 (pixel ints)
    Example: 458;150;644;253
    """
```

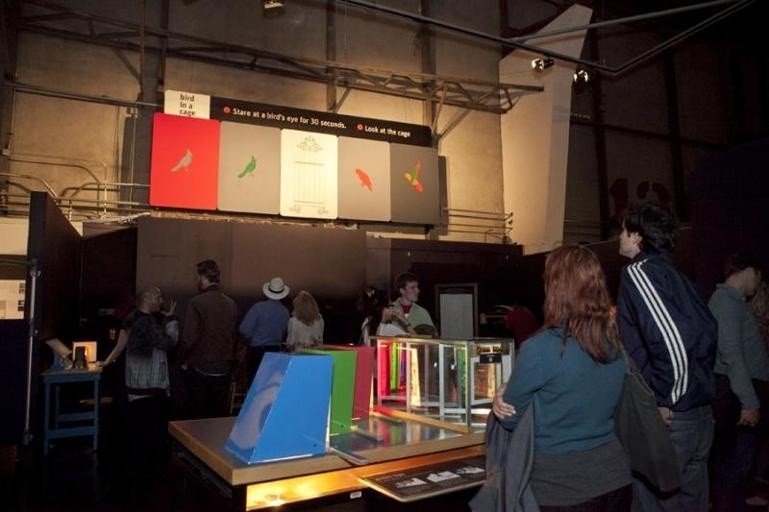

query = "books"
374;333;522;413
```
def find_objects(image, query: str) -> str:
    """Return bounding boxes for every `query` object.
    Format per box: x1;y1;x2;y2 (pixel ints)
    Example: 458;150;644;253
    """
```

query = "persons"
619;203;723;511
494;304;538;349
285;289;325;346
746;278;769;506
376;272;437;339
466;244;684;511
93;284;182;402
706;250;768;510
360;296;420;345
176;258;237;415
238;278;291;390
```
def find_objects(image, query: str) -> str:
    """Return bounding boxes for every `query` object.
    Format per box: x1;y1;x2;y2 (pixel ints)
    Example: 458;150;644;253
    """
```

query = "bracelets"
405;322;411;328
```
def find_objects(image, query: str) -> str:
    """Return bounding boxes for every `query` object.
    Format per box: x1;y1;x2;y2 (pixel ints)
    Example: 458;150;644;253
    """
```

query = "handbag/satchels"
613;365;678;487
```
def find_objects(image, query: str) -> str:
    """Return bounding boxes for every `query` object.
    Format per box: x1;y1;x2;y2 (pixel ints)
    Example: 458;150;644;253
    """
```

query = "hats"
262;277;290;300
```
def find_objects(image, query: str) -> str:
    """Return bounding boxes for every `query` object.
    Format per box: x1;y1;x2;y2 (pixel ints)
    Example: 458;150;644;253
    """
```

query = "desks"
40;364;100;455
168;404;488;512
